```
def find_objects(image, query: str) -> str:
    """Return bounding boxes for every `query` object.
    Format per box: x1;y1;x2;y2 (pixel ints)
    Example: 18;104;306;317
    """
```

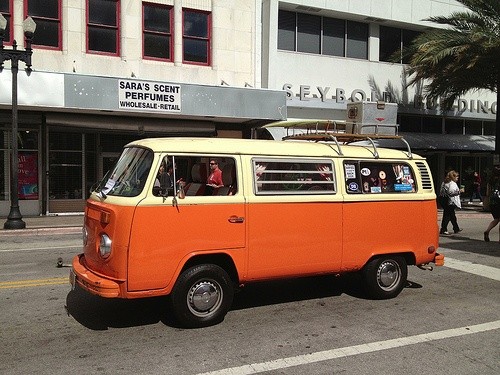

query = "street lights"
0;11;38;231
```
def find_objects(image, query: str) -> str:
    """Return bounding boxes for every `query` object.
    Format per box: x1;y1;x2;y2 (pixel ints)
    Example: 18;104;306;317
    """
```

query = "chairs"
212;165;235;196
185;163;206;196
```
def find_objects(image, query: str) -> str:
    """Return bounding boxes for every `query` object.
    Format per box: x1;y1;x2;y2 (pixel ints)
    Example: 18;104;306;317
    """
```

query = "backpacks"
439;185;450;205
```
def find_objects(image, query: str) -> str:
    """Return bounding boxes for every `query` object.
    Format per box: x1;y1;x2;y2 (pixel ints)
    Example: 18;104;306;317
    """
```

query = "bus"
67;118;446;328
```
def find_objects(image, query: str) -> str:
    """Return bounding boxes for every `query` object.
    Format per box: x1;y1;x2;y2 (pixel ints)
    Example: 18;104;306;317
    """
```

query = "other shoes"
439;230;450;235
454;228;463;234
484;232;490;242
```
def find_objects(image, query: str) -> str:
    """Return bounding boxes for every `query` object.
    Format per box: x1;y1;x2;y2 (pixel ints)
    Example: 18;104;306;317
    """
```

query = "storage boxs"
346;101;399;134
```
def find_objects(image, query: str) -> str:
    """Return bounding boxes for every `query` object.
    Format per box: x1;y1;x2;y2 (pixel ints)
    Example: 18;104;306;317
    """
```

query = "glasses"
210;163;216;165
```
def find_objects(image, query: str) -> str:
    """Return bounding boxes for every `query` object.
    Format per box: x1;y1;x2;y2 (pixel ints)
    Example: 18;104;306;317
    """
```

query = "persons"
138;157;222;196
440;169;500;242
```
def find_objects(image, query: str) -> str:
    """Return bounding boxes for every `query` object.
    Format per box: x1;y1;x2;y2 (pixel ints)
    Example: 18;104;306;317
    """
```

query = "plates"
206;184;217;186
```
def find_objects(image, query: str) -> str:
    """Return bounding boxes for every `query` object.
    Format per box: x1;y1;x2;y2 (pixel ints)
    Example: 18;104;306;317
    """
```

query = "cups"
209;178;213;184
460;185;465;192
